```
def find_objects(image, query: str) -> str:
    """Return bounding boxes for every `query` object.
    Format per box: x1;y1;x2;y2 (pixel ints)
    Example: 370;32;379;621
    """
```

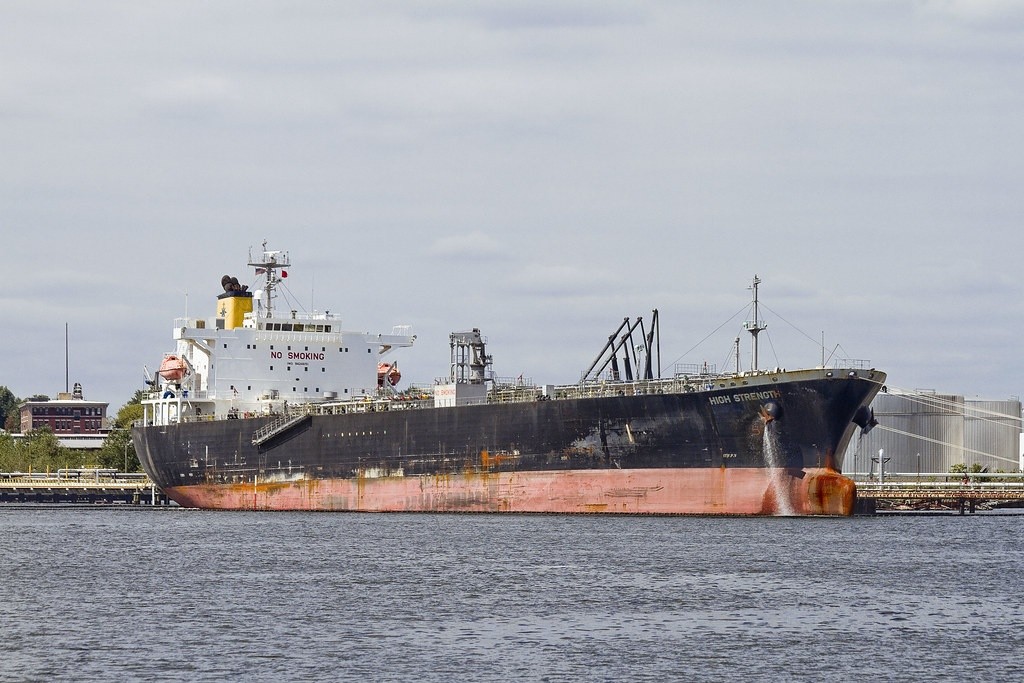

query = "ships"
126;238;891;521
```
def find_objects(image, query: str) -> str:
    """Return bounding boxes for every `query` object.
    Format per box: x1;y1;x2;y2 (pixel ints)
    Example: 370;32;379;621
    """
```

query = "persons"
228;406;239;418
268;403;274;414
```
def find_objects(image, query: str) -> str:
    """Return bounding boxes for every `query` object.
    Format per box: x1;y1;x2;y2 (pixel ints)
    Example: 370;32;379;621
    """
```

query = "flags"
281;271;288;277
256;268;266;275
